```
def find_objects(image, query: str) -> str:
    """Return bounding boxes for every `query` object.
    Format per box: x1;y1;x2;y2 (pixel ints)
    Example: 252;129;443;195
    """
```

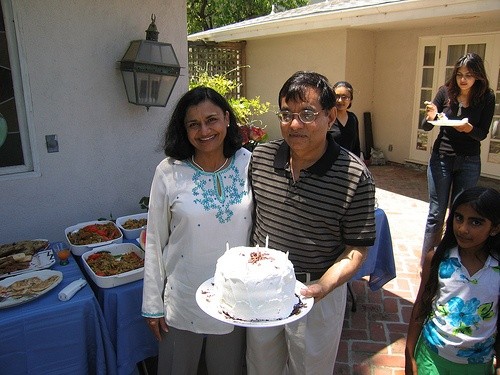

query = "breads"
0;240;48;274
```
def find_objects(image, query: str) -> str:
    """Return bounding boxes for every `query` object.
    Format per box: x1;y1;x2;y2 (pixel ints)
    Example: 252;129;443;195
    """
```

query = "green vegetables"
67;226;103;245
93;250;125;275
121;219;140;230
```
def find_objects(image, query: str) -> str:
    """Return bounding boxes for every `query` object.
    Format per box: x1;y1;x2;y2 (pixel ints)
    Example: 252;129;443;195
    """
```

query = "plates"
196;277;314;328
0;239;63;308
426;119;464;126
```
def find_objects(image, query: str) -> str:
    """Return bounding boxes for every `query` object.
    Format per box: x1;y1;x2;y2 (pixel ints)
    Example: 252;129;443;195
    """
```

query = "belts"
295;272;326;282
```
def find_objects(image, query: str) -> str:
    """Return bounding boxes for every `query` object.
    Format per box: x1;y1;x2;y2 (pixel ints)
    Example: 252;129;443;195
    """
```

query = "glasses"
336;96;351;101
276;105;328;124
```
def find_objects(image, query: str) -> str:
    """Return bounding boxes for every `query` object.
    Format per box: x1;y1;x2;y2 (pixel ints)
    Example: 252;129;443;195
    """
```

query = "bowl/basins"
64;213;147;289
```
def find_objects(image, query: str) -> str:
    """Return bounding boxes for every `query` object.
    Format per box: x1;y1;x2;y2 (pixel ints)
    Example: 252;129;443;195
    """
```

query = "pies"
0;274;60;293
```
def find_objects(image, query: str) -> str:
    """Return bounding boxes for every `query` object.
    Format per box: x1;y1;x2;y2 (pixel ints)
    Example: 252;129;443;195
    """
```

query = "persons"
142;85;252;375
420;52;496;274
328;81;361;158
250;70;376;375
405;186;500;375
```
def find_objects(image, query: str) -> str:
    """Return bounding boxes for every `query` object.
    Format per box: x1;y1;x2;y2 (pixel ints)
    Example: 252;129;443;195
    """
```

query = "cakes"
214;246;296;322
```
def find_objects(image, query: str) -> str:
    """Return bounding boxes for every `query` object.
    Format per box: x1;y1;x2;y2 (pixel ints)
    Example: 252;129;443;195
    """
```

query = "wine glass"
53;241;73;266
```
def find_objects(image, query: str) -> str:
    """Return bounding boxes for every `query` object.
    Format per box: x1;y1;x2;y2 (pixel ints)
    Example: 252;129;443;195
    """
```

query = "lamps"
119;13;183;112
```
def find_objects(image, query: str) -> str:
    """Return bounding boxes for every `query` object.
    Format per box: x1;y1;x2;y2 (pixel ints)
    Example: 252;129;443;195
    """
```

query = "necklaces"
193;158;228;173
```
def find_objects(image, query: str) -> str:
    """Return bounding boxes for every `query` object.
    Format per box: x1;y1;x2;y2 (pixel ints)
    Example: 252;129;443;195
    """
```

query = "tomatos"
86;225;108;236
87;254;106;276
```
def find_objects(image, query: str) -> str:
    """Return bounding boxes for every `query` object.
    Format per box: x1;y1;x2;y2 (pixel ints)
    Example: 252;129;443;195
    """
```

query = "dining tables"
0;209;396;375
